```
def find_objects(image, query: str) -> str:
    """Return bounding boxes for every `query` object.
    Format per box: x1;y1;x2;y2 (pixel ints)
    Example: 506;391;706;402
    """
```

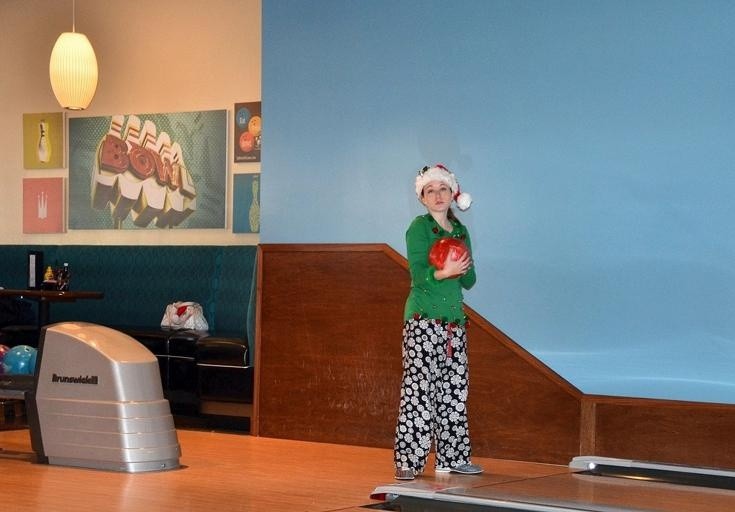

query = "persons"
393;165;486;480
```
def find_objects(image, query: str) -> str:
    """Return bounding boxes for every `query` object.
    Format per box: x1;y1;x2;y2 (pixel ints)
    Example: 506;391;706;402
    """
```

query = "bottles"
44;266;55;280
57;263;72;292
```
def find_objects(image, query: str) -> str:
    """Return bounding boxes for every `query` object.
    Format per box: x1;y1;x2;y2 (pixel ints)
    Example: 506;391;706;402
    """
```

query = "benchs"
0;244;259;413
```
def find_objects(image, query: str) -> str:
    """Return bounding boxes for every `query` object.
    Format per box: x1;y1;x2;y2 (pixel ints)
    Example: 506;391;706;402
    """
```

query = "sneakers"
394;469;416;480
436;464;484;474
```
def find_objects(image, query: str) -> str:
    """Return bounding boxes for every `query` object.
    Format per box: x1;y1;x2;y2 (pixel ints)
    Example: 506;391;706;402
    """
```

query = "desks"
1;287;104;342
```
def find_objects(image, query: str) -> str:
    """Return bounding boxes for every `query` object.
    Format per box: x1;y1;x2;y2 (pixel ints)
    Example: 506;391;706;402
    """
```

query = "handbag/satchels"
160;301;208;332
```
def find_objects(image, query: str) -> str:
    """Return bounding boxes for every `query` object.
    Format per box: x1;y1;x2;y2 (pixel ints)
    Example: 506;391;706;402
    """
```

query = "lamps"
47;0;100;111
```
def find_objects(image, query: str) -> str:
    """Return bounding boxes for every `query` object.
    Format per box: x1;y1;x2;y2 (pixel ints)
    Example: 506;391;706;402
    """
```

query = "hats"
416;165;472;211
